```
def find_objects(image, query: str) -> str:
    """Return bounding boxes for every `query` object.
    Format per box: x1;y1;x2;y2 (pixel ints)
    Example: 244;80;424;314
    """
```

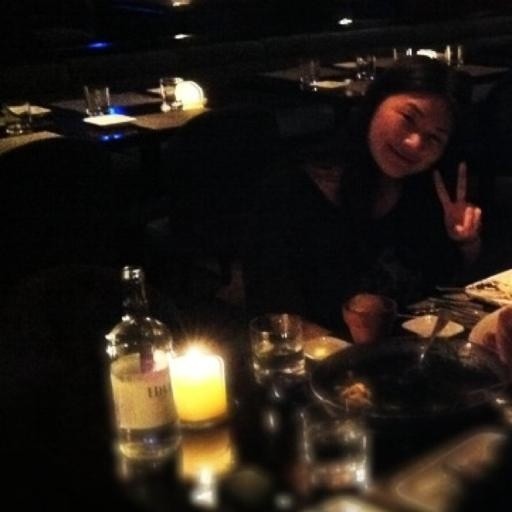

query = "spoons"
404;309;454;376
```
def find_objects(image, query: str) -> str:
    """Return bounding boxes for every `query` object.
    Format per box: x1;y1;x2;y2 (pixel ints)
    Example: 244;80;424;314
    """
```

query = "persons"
250;52;492;329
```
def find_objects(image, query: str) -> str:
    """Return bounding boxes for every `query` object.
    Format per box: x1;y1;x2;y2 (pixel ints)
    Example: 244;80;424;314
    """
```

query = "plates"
309;341;511;422
301;334;350;362
402;313;465;343
3;104;52;121
82;114;136;128
310;81;348;93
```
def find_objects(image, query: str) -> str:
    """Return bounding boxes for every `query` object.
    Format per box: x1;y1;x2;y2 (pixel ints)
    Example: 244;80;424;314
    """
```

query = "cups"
159;77;184;113
248;312;306;379
343;293;398;347
393;44;416;72
356;53;377;81
5;101;33;136
444;42;466;68
300;400;370;495
84;84;111;116
297;56;321;92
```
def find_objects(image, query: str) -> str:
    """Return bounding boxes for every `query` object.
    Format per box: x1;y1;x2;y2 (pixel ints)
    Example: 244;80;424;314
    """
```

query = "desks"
82;270;512;512
0;92;210;157
260;54;512;102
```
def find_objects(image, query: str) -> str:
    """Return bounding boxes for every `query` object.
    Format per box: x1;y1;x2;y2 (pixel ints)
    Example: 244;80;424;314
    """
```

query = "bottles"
104;267;182;465
